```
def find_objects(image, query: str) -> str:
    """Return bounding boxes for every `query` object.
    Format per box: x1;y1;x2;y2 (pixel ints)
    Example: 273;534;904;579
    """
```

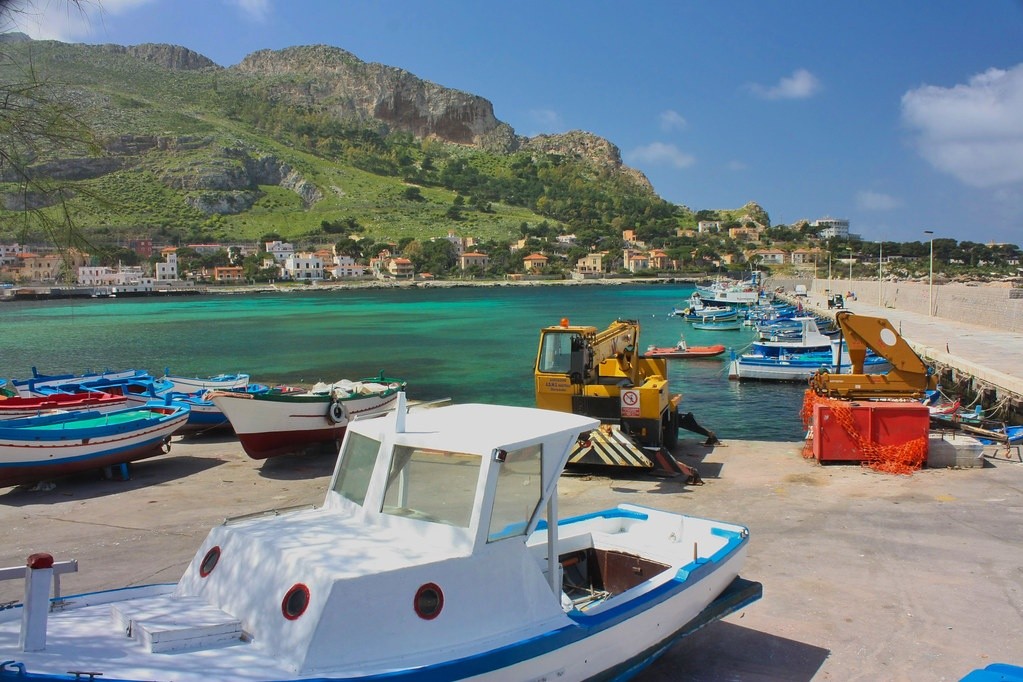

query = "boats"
973;424;1023;447
869;384;943;407
926;398;962;416
202;369;408;461
0;363;306;431
672;263;934;391
0;390;191;480
929;405;1006;432
0;381;765;682
643;334;726;359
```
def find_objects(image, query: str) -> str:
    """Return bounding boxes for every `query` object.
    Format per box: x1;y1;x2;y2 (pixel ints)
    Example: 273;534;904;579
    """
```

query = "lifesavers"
330;403;346;424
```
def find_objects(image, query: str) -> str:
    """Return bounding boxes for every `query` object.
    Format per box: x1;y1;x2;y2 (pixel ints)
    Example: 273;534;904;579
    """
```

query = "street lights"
874;241;884;307
825;251;832;296
924;230;935;316
846;247;853;292
814;254;817;279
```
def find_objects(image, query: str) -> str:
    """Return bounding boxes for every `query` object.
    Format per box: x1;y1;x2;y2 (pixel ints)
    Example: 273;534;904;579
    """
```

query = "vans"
795;285;808;297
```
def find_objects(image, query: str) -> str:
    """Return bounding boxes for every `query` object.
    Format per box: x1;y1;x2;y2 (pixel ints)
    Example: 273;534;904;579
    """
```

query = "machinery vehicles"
532;317;730;487
